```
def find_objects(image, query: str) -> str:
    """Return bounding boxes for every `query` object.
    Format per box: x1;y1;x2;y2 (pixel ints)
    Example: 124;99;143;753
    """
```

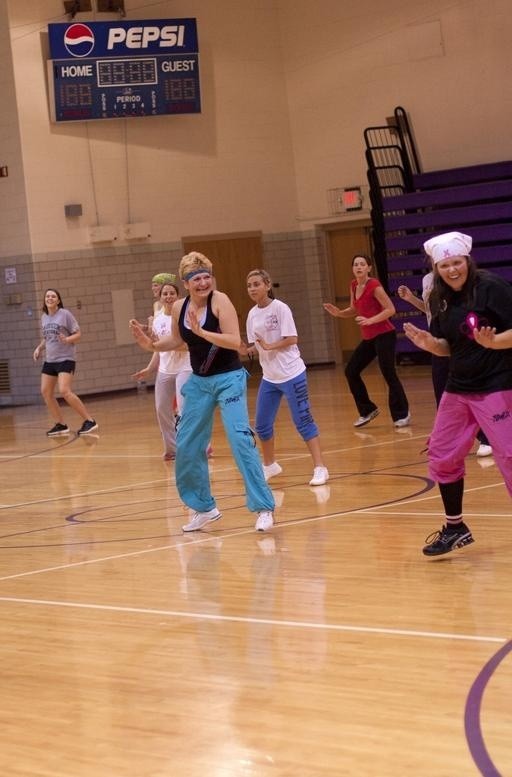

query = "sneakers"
353;409;380;427
255;511;273;531
394;414;410;427
477;445;492;456
164;452;176;460
46;423;70;435
78;419;98;433
182;508;222;531
207;448;215;457
423;523;475;555
310;466;329;485
262;462;282;481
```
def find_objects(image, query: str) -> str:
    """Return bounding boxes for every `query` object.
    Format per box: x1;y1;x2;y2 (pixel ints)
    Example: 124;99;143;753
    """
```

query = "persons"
401;231;512;556
131;272;178;417
322;255;412;427
33;288;99;434
245;269;330;486
398;258;494;457
132;251;275;533
130;284;214;462
